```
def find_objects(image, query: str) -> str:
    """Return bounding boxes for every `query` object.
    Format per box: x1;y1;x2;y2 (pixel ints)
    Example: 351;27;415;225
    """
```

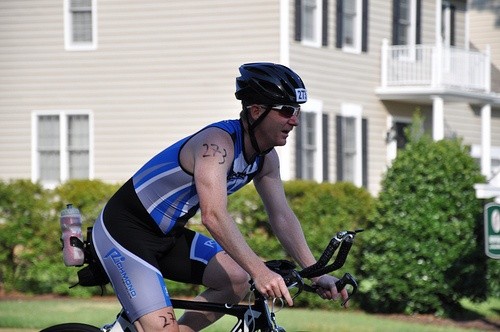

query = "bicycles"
38;224;365;332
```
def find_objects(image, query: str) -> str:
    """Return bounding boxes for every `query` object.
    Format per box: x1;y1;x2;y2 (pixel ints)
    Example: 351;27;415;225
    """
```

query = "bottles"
60;204;85;266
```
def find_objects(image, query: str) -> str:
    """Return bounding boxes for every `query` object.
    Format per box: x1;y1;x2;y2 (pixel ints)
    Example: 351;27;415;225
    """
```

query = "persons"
92;62;350;332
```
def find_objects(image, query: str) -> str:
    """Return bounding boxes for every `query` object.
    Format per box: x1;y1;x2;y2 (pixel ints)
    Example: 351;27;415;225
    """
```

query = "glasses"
245;103;301;119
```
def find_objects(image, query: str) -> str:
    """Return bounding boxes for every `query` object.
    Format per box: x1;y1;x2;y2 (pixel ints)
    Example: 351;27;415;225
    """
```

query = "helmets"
235;61;308;105
